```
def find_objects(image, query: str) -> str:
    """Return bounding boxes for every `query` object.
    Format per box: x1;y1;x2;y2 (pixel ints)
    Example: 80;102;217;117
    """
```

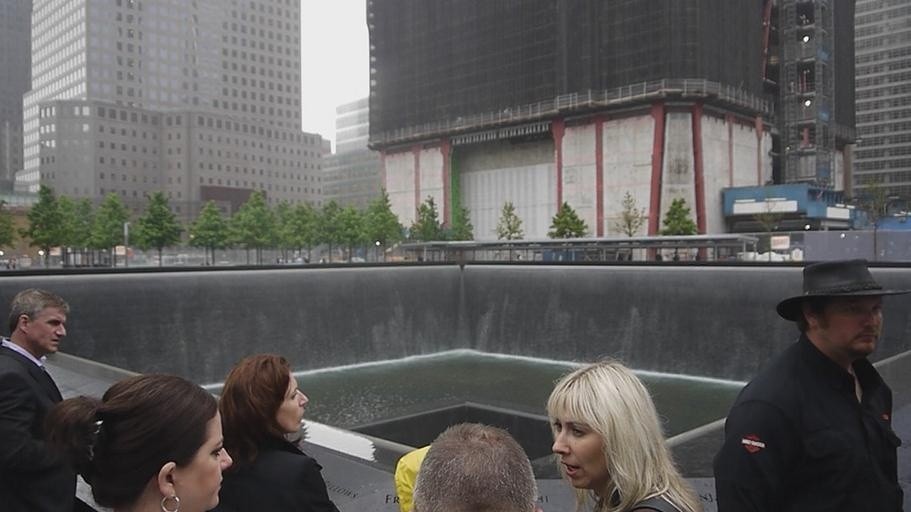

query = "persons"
408;419;545;511
715;256;910;511
545;354;702;511
217;352;338;510
0;288;96;512
35;371;235;512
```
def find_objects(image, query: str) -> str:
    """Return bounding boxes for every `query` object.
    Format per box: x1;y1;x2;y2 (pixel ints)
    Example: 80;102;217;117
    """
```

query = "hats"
776;257;911;322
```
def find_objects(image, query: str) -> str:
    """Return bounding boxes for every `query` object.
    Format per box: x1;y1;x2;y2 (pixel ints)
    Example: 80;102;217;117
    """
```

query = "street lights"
374;238;382;267
122;219;131;266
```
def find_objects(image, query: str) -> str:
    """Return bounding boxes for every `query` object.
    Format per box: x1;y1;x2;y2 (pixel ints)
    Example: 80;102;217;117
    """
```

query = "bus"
154;253;211;266
